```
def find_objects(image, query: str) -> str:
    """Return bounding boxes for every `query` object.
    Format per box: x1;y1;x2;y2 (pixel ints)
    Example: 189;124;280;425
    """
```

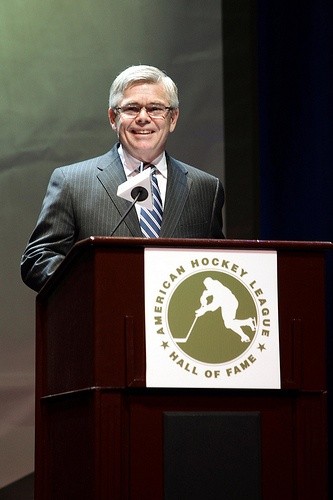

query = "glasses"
115;103;174;118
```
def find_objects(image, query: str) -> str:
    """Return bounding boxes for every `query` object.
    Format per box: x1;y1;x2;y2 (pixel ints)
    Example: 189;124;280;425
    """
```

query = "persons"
18;65;225;294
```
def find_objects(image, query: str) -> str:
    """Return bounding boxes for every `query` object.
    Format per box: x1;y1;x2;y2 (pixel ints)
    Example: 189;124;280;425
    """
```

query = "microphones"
109;166;153;236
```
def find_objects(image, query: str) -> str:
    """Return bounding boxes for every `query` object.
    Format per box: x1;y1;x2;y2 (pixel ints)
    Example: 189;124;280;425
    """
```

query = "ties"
136;164;163;237
